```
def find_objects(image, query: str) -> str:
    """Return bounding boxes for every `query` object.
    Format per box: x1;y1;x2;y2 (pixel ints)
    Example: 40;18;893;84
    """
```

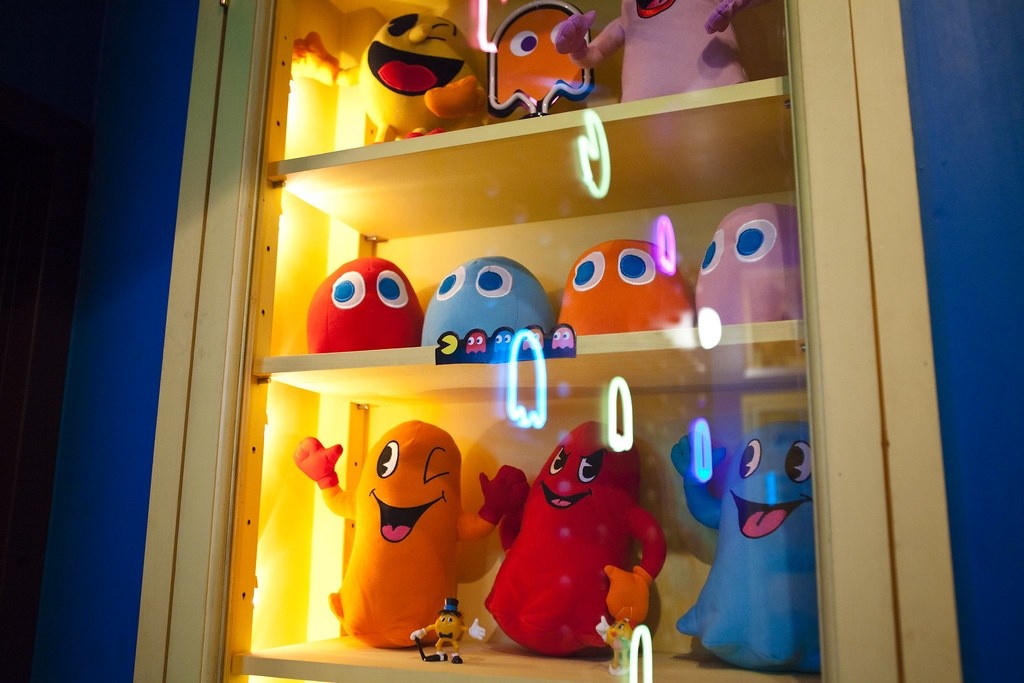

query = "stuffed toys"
557;238;694;336
670;421;821;672
549;0;750;103
421;254;552;347
481;421;666;657
305;257;424;355
694;201;804;326
293;420;529;651
291;12;487;146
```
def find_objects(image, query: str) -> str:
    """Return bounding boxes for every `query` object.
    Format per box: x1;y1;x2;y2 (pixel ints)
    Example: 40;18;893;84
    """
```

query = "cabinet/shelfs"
219;0;902;681
